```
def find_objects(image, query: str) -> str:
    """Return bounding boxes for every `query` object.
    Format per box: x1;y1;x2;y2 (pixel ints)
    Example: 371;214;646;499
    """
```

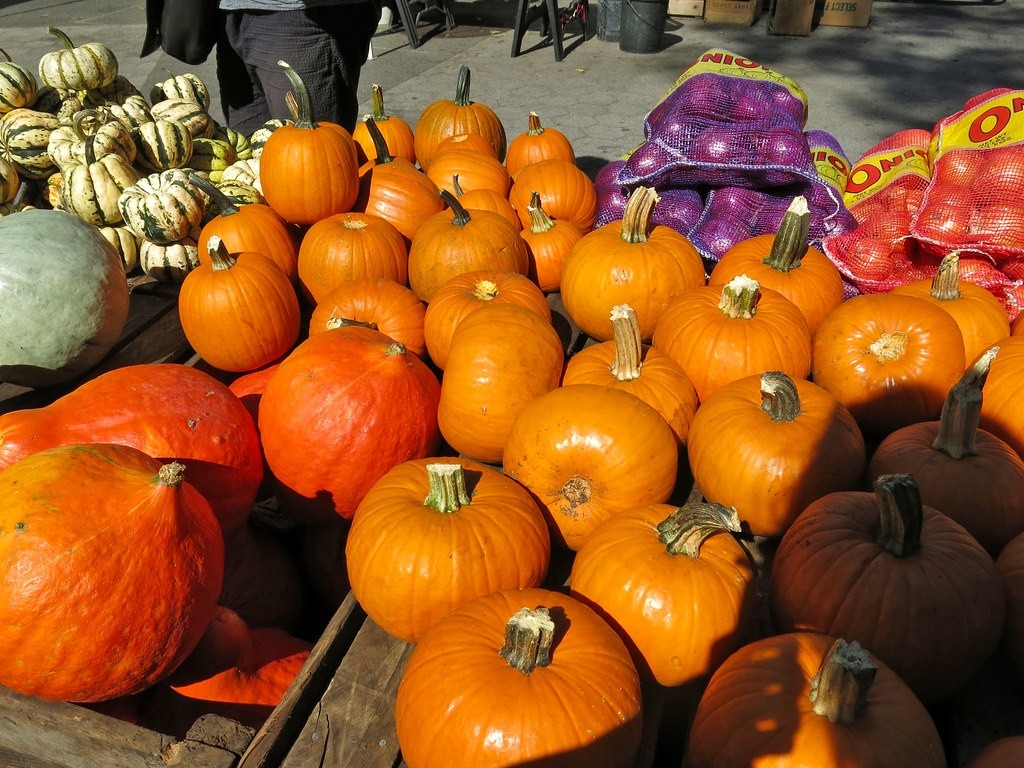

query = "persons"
139;1;383;138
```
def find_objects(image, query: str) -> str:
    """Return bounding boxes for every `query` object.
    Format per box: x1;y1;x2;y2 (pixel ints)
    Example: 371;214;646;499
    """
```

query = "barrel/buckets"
595;0;622;43
619;0;670;55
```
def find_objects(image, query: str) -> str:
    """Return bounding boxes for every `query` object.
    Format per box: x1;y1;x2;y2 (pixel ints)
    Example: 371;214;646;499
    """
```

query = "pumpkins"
0;25;1024;768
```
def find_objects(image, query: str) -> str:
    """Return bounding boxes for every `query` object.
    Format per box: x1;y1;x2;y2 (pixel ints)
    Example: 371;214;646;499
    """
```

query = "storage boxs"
705;0;872;37
